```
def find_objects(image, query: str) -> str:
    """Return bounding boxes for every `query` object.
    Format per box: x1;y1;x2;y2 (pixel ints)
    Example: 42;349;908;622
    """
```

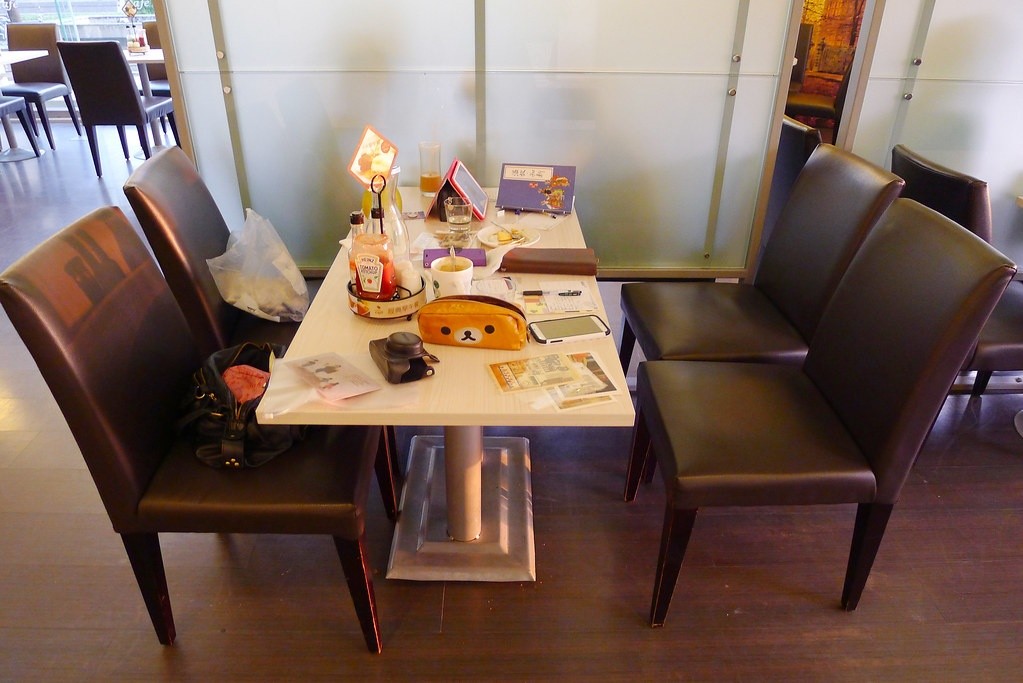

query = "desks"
0;49;50;162
236;185;635;583
121;50;168;160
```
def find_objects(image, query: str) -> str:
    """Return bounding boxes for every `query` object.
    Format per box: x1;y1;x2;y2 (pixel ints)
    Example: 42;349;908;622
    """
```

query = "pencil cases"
416;295;531;350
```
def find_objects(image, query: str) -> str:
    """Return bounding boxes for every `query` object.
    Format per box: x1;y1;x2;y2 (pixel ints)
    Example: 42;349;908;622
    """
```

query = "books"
501;247;597;276
495;162;577;214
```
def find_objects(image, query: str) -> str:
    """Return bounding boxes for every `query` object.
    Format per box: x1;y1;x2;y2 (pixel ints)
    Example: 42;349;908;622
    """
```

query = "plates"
477;224;540;247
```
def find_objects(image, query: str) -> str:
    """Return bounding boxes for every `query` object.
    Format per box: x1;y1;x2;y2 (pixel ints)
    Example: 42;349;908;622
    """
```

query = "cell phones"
529;313;611;346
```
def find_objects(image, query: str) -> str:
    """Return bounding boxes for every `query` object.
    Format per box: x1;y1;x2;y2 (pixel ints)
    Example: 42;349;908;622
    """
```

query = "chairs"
786;50;854;144
3;206;397;663
789;22;814;93
614;143;906;485
131;21;171;134
892;143;1023;398
57;40;180;180
123;144;402;515
759;116;821;248
0;87;41;159
636;198;1015;632
4;23;81;149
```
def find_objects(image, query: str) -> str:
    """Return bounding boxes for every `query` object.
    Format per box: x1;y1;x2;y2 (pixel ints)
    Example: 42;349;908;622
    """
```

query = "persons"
831;54;855;146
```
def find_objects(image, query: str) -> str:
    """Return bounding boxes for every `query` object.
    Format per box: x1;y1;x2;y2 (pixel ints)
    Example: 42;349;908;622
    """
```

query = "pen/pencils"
514;210;521;217
523;289;582;296
544;212;557;221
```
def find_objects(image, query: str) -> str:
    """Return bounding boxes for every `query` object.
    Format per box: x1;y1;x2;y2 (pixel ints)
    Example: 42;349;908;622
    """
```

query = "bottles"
349;165;422;300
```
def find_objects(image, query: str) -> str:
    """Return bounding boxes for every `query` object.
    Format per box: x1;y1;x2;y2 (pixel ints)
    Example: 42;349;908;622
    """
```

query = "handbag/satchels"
175;341;307;466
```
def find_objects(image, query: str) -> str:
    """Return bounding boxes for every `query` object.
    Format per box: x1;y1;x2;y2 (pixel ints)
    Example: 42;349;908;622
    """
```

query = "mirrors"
739;0;886;283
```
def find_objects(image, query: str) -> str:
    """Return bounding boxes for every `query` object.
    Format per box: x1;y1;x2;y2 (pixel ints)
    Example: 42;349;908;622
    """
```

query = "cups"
475;277;516;304
419;141;442;198
444;197;474;242
430;256;473;299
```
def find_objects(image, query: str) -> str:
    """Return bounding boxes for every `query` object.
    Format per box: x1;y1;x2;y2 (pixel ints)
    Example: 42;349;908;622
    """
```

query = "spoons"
491;221;521;239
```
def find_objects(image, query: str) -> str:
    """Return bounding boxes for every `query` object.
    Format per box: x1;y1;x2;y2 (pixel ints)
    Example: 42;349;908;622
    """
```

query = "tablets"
424;160;489;222
